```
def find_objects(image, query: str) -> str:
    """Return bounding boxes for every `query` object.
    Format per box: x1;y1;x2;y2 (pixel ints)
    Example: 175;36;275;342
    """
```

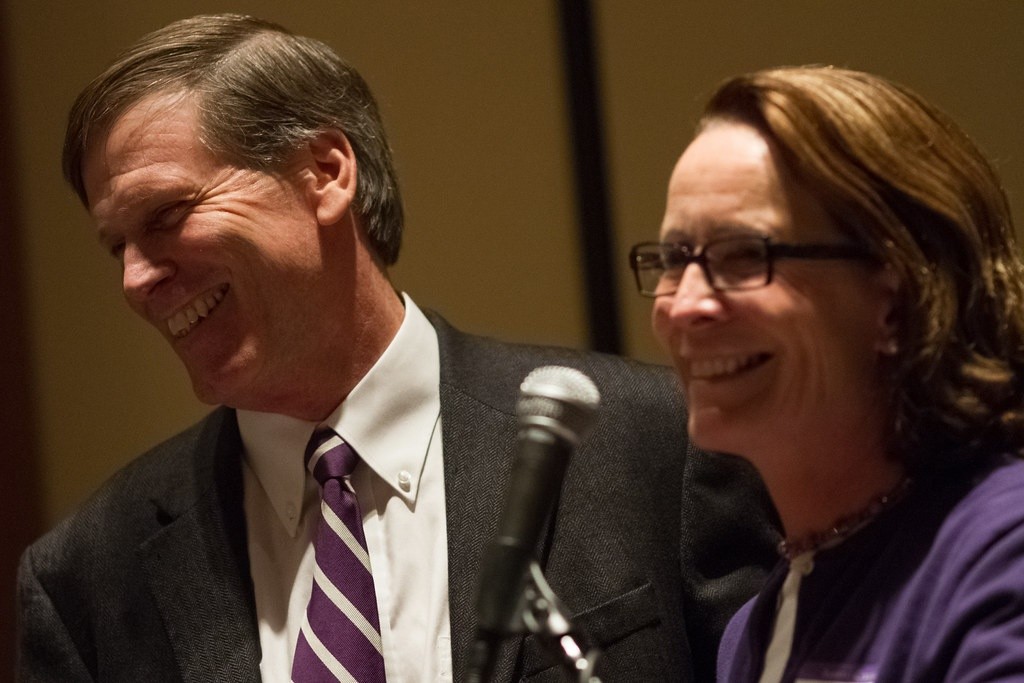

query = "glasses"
627;232;872;297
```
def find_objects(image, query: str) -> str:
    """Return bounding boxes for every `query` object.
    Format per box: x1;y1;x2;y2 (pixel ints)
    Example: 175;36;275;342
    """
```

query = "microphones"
461;366;601;683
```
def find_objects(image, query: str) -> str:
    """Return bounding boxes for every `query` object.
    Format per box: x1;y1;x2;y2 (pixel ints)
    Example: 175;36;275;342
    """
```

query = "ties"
291;424;385;682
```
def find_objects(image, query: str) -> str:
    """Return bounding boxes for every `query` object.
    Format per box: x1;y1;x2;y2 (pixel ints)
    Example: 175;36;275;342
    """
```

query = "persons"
629;64;1018;683
11;11;793;682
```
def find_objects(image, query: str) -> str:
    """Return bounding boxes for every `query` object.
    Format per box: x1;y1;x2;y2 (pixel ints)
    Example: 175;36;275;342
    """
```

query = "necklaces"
770;487;904;559
